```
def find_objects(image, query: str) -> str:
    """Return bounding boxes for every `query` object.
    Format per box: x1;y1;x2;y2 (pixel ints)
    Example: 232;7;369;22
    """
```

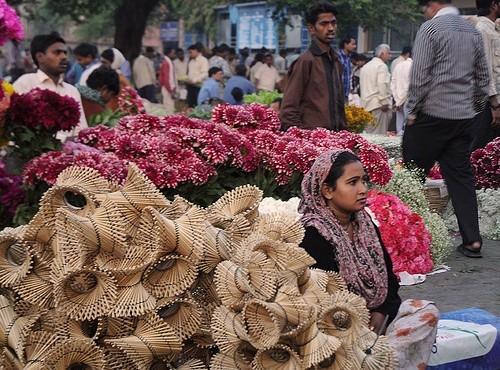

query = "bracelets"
491;106;499;110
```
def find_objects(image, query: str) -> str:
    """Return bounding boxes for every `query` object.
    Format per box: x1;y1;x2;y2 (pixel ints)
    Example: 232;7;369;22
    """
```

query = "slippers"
457;244;483;257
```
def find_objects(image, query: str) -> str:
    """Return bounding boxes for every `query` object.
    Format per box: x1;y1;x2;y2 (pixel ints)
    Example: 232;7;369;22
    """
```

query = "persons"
280;3;349;133
401;0;500;258
77;66;119;128
5;0;500;124
254;54;283;94
225;64;255;105
298;148;440;370
335;35;356;102
12;32;89;143
360;44;394;134
391;48;414;134
197;67;224;106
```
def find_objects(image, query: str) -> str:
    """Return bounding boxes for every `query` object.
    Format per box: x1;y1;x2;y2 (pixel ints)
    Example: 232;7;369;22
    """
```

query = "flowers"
0;1;500;286
